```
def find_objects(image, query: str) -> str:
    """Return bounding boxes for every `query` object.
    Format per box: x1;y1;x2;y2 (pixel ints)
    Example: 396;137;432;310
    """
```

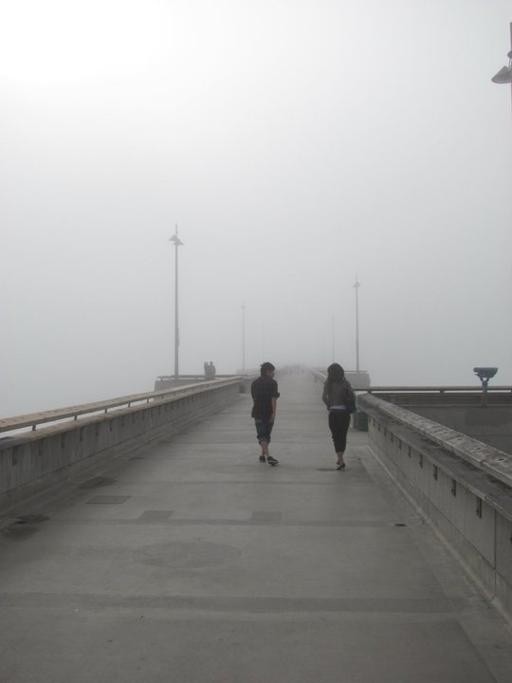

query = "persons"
207;360;216;377
321;362;355;471
249;361;281;464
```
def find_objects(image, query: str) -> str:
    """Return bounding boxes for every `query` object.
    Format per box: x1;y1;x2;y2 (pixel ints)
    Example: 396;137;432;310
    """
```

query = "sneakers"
267;457;280;465
259;456;265;463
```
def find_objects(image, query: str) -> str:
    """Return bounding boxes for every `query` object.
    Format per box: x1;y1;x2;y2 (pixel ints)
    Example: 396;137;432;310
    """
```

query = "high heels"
336;460;345;471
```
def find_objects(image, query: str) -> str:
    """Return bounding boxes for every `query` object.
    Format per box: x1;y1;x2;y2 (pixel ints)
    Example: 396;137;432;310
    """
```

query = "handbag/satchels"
344;389;356;414
